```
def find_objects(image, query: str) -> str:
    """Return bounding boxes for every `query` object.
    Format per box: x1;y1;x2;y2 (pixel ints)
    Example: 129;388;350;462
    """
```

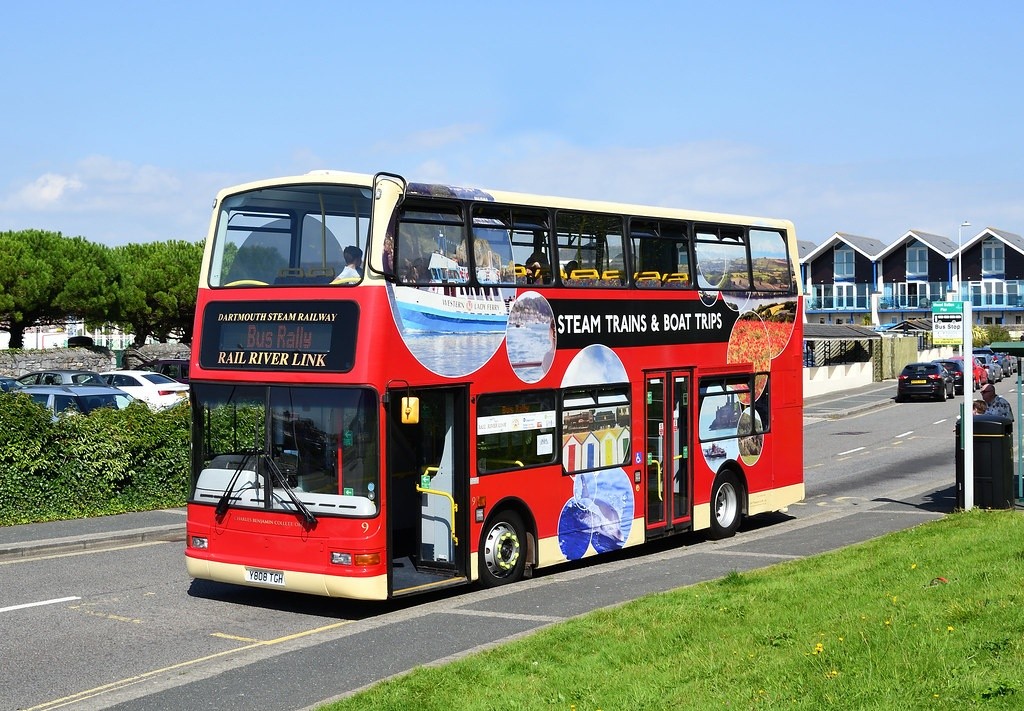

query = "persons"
327;247;364;285
407;257;432;284
974;383;1014;422
564;260;578;280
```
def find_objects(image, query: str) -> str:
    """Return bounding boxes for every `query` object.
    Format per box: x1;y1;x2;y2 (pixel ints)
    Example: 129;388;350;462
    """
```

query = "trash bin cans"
953;417;1017;510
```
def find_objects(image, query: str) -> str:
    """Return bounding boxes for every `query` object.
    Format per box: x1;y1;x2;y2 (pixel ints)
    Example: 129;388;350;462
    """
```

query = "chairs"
107;375;123;385
503;267;689;288
89;398;101;409
45;375;55;385
225;268;363;286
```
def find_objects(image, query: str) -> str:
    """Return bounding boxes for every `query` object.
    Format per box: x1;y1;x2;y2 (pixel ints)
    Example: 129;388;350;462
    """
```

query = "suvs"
133;359;190;384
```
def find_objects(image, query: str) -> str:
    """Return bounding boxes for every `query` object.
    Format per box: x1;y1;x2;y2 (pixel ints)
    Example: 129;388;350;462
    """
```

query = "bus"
180;171;806;601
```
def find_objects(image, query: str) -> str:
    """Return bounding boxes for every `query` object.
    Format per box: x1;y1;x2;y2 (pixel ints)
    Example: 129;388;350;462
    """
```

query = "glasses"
980;390;991;394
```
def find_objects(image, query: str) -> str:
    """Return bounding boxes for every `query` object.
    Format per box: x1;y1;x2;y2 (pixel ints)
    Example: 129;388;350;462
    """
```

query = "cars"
1;374;24;395
79;370;190;414
14;385;138;421
15;370;108;388
897;348;1018;402
